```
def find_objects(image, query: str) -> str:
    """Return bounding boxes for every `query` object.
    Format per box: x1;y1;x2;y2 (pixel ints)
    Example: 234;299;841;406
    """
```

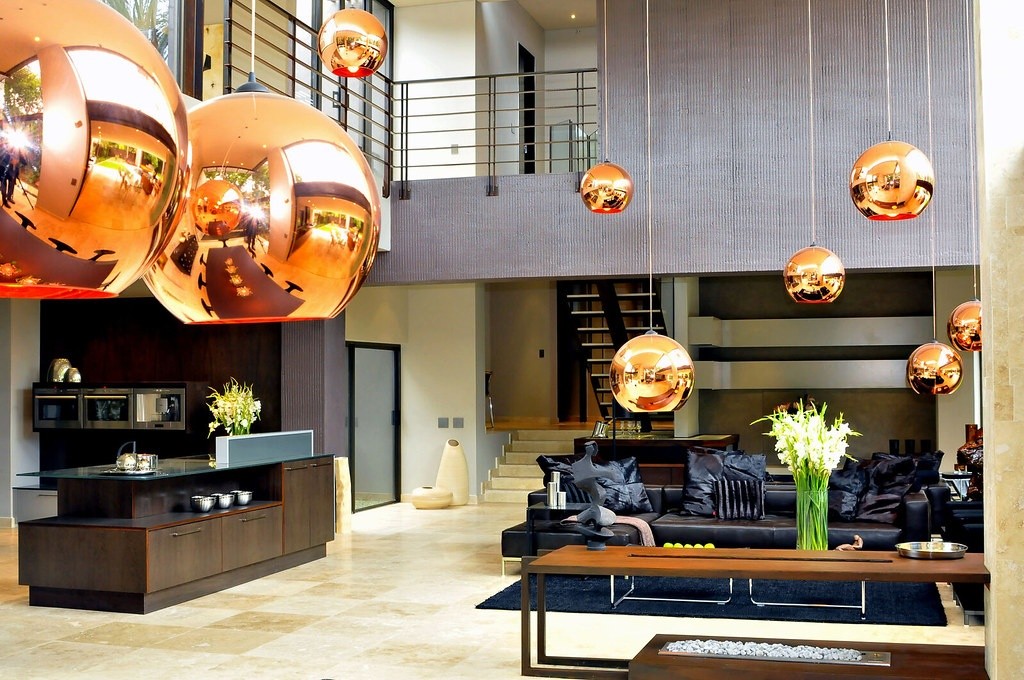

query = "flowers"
205;377;262;438
750;393;863;550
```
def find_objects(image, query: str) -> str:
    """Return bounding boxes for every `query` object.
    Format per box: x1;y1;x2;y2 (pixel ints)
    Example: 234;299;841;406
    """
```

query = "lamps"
0;0;982;414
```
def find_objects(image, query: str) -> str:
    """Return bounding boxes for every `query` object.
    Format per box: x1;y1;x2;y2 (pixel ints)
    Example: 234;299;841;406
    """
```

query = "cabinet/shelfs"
11;454;336;616
141;174;154;196
347;233;355;250
34;382;188;431
612;384;620;395
170;235;198;277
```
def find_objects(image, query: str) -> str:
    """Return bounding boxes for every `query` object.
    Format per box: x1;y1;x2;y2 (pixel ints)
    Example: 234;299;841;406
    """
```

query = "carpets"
477;570;948;628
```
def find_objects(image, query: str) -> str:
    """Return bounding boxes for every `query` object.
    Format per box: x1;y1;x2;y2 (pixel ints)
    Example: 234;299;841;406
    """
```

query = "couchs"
913;374;950;394
501;483;984;628
799;287;830;300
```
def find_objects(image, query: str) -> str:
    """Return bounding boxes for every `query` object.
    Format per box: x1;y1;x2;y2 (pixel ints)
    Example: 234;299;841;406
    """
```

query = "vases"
957;423;984;498
795;490;829;551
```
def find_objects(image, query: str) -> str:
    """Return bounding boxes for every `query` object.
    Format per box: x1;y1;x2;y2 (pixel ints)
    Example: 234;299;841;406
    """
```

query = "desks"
0;206;118;290
942;471;974;502
519;544;988;680
527;500;594;577
206;246;304;317
635;389;677;410
575;429;740;486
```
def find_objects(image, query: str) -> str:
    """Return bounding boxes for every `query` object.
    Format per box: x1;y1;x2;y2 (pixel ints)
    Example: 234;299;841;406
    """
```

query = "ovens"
34;389;186;430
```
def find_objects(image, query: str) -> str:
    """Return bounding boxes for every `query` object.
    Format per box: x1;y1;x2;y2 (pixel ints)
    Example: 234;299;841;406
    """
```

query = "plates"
895;542;968;558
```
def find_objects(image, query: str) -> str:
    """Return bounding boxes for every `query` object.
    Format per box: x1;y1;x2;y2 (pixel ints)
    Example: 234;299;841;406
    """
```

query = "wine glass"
619;420;642;438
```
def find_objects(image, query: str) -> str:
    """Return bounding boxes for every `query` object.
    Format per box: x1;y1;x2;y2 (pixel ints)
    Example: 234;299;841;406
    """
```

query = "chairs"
16;211;120;292
484;371;495;427
198;237;305;318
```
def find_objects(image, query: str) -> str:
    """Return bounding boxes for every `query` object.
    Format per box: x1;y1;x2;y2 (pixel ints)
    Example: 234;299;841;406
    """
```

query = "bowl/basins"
189;495;217;513
230;490;254;505
211;493;235;510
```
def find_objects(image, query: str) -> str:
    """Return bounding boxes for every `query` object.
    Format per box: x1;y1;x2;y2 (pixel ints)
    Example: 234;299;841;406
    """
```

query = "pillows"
537;442;944;523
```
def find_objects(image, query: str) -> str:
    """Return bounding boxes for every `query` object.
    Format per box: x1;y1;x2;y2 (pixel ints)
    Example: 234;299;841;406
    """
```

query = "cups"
931;533;943;552
959;465;967;473
